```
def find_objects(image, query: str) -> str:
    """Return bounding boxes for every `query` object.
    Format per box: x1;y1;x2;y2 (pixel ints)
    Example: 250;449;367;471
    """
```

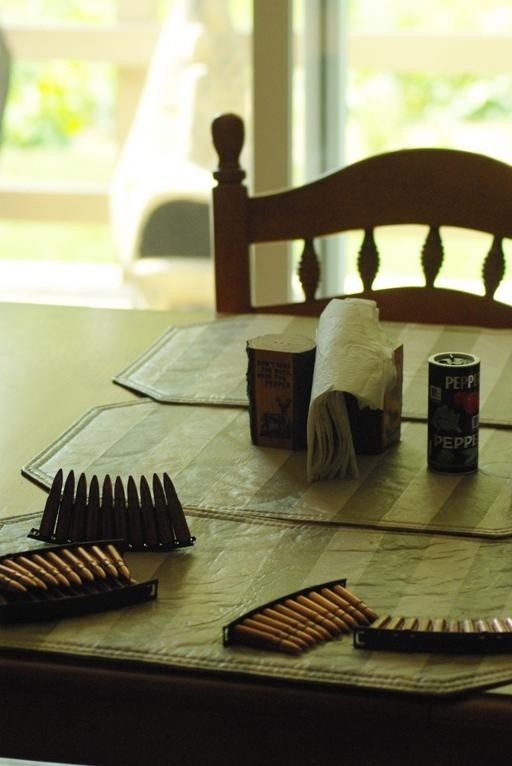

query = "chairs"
211;114;512;330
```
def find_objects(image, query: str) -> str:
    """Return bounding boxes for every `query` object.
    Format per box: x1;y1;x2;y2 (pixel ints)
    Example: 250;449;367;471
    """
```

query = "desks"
0;301;512;766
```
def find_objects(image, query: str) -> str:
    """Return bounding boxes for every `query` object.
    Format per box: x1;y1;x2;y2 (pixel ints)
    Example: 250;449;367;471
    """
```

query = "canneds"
426;349;483;477
